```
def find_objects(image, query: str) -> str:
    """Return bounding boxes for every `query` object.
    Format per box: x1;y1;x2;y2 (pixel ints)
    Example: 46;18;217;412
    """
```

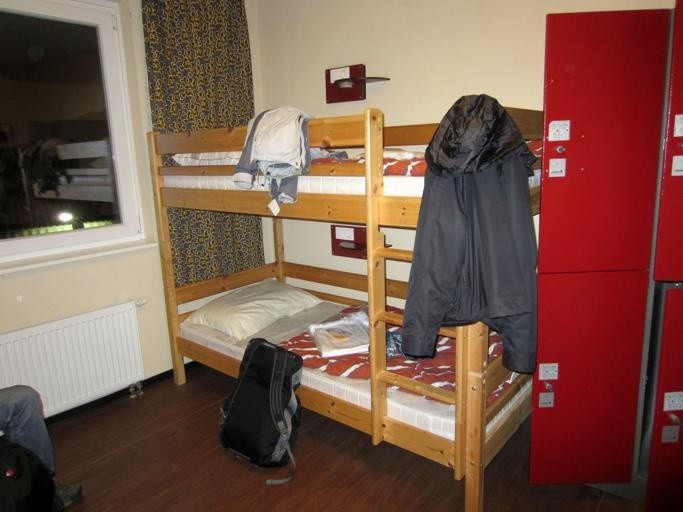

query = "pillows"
185;277;323;341
172;151;242;167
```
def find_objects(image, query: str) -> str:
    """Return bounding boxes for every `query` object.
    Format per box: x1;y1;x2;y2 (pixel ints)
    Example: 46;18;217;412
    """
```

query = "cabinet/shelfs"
637;0;683;512
527;7;672;488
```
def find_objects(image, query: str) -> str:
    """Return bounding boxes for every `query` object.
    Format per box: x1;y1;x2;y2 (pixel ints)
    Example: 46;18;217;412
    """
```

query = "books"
310;318;369;358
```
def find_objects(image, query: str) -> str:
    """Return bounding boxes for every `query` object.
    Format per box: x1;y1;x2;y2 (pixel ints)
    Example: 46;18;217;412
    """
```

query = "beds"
19;140;115;210
146;107;544;512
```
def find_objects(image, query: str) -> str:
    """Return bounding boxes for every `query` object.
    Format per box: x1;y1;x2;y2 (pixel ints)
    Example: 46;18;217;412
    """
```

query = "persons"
0;387;82;509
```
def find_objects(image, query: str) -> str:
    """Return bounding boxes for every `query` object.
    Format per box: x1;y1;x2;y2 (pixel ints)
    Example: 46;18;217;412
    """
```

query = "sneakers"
54;486;82;510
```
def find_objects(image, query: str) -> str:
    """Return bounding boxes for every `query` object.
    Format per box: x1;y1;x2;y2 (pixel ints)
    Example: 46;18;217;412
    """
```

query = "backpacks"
217;337;303;484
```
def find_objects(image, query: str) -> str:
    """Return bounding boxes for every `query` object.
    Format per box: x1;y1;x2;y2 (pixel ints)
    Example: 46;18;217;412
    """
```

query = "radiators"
0;300;146;419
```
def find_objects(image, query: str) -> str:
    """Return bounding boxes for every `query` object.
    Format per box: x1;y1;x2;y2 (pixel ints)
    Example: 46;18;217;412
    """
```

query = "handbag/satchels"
1;441;61;511
